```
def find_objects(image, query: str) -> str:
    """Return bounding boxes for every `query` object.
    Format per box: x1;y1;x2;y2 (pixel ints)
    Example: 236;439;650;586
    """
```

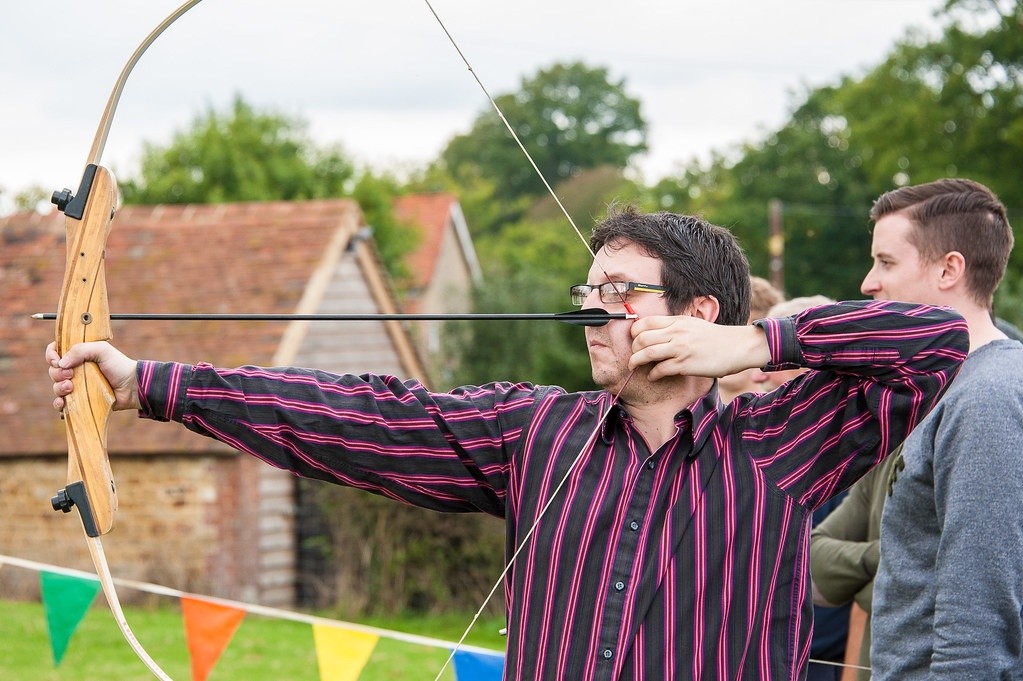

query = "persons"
861;178;1023;681
751;295;837;396
811;445;904;681
45;204;969;681
715;274;783;407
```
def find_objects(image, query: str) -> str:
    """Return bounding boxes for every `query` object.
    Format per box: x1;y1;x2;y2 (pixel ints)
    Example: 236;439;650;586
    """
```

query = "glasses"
570;281;670;305
